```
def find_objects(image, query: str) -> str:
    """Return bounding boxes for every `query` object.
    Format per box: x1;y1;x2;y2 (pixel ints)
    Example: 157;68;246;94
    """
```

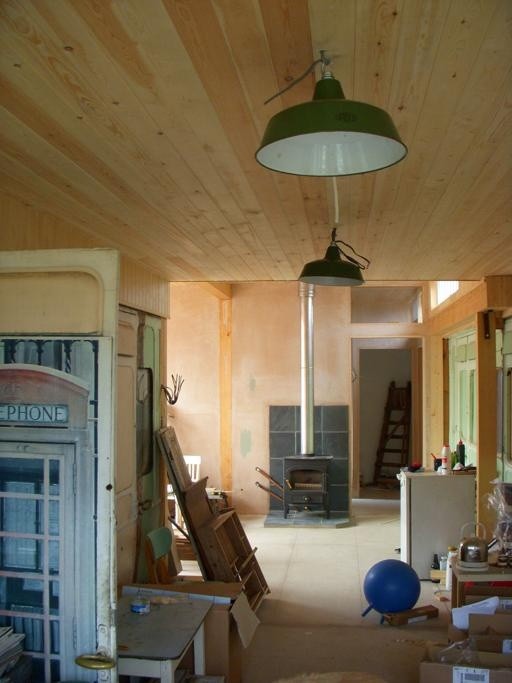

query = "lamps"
252;48;411;288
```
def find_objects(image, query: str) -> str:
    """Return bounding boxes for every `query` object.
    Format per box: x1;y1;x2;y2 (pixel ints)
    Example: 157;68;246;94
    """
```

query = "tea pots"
458;522;498;562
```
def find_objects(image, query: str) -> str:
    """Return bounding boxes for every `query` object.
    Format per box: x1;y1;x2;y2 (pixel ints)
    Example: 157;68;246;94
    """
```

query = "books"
0;626;26;678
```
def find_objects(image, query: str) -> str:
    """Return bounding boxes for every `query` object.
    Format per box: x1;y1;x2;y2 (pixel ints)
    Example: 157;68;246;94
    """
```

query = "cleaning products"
442;445;451;469
457;440;465;465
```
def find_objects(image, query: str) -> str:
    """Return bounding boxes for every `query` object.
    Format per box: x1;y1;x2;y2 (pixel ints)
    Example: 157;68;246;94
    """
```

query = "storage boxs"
417;585;511;683
121;580;261;683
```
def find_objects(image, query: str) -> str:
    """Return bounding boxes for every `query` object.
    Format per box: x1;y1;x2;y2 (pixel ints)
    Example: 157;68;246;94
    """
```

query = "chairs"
183;455;202;483
145;527;204;582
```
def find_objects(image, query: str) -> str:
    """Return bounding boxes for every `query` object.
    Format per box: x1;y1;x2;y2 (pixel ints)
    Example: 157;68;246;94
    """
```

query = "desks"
115;594;212;682
167;488;224;529
449;558;512;609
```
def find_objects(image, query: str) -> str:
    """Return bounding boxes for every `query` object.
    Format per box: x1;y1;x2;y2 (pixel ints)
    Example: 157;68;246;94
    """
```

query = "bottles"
456;438;465;467
431;546;458;592
442;446;451;475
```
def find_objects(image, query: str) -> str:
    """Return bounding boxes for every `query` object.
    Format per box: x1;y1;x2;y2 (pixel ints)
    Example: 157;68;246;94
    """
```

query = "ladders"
373;381;411;487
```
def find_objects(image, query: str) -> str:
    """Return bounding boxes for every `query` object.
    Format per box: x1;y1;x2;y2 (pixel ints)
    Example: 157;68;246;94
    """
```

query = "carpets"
240;622;450;681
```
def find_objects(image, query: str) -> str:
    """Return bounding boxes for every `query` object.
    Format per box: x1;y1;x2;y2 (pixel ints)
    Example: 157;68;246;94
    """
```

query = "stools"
383;605;438;626
